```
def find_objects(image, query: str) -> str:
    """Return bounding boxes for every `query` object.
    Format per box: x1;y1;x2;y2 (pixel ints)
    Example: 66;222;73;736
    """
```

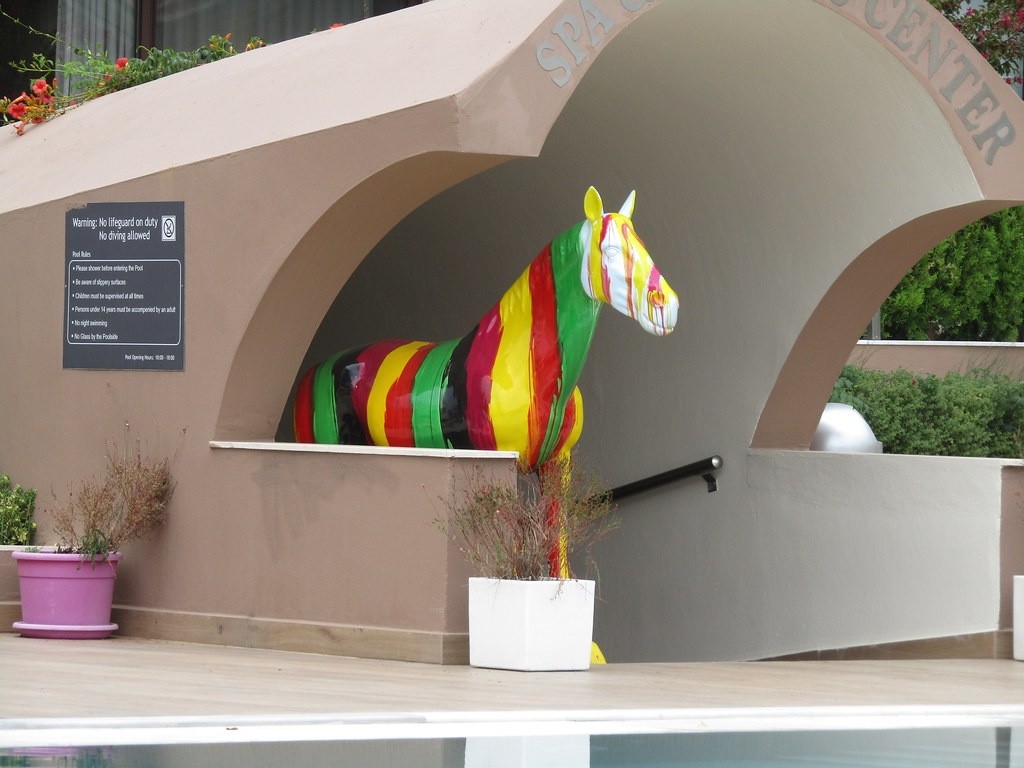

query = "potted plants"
422;448;624;673
12;418;187;639
0;474;78;633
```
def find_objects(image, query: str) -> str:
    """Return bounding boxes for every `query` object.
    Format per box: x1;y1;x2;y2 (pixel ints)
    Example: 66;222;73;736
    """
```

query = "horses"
291;183;681;667
